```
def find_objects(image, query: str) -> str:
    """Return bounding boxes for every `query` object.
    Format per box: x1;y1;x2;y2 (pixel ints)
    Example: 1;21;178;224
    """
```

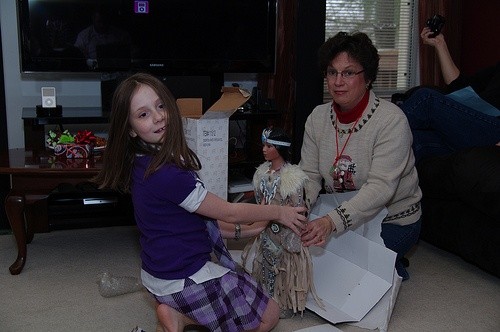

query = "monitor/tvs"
16;0;278;75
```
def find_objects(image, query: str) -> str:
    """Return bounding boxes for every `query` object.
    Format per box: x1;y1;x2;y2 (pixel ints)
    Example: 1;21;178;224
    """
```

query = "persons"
73;6;123;71
297;32;422;281
89;73;309;332
399;27;500;154
241;126;327;320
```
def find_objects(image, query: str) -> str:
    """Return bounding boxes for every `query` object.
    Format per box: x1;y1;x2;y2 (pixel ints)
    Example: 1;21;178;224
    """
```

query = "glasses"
326;67;365;78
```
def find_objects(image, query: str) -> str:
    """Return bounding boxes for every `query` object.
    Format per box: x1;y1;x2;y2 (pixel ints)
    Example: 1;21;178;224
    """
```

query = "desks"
0;148;107;275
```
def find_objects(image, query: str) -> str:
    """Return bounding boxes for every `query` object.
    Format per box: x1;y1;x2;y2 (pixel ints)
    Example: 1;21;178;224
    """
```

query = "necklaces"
335;94;369;174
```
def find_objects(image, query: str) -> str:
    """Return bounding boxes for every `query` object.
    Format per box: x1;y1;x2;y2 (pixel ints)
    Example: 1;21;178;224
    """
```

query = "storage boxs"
55;142;93;158
176;87;252;203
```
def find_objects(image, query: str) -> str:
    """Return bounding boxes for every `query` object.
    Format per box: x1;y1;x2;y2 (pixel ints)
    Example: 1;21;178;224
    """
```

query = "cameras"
426;14;446;38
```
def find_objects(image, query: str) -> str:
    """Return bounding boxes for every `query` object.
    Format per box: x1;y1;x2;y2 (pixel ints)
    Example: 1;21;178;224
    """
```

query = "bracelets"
233;223;241;240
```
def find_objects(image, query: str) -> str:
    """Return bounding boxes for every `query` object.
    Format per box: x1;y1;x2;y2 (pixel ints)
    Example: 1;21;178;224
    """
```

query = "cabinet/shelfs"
22;99;285;228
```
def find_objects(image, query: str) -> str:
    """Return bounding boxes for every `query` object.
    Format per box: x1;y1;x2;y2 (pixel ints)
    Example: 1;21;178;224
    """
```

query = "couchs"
392;74;500;279
